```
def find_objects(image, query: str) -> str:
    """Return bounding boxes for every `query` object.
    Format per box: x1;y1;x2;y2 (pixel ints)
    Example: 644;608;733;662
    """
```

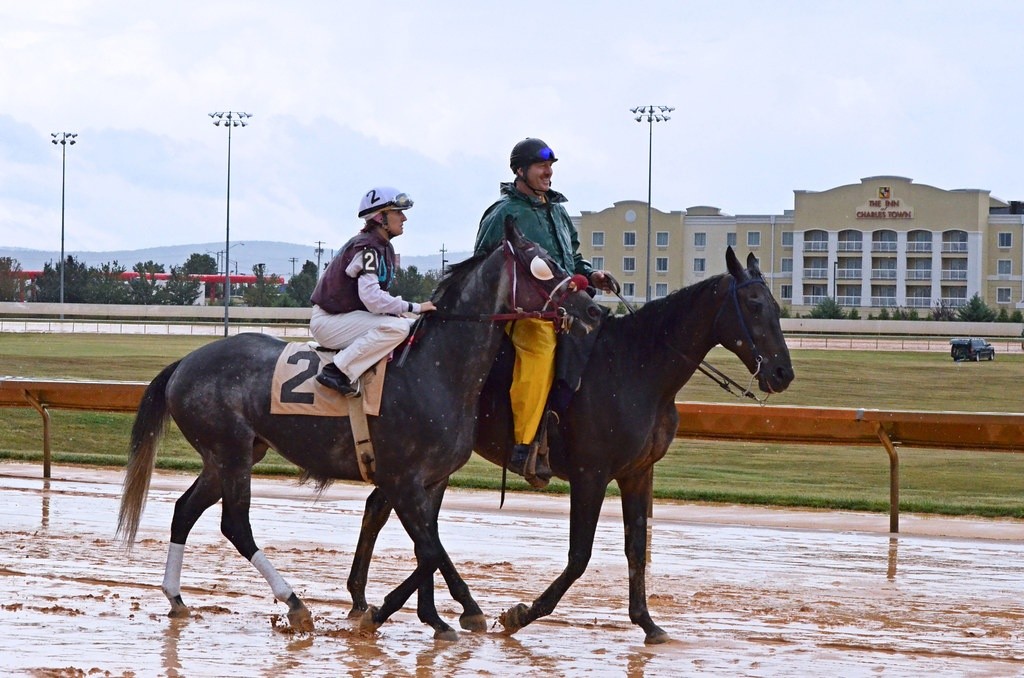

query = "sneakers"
506;445;551;478
314;363;360;396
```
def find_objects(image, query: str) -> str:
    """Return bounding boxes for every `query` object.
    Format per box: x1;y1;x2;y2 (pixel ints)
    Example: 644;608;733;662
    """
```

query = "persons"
308;184;439;399
474;135;614;481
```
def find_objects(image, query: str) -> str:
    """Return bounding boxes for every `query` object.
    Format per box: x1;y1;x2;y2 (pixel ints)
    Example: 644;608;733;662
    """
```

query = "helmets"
357;188;413;219
510;136;556;171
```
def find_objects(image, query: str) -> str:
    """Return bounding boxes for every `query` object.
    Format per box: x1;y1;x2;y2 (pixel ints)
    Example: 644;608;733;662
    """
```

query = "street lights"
207;110;254;338
628;105;676;304
49;131;79;319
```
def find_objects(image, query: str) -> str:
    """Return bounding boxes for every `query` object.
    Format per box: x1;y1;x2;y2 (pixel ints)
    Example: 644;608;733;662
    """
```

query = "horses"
291;245;796;645
114;215;602;643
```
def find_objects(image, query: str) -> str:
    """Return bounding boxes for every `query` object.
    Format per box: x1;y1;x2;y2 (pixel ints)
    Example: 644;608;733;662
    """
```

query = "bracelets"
408;301;414;313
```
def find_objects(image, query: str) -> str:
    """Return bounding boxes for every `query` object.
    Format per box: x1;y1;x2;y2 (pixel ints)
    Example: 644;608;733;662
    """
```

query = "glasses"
394;193;412;207
533;146;554;161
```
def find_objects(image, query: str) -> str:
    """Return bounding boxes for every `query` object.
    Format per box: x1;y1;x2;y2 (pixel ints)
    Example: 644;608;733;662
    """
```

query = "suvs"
949;337;995;363
